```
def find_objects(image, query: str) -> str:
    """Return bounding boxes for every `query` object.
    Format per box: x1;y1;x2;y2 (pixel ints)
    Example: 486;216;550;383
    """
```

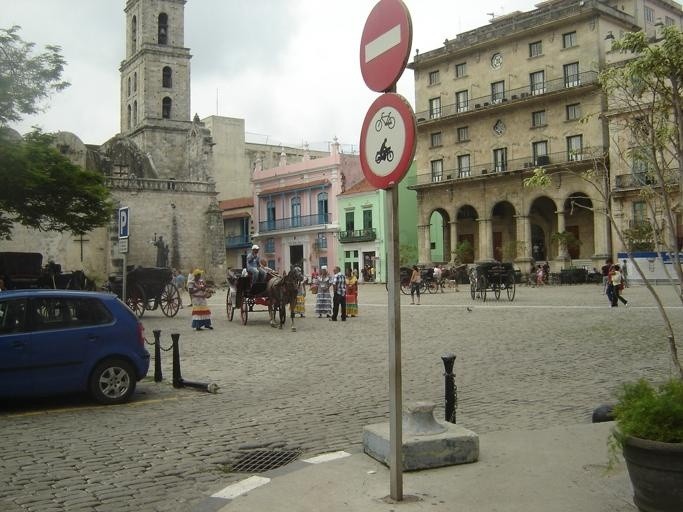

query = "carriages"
465;259;521;302
222;263;307;337
385;264;458;295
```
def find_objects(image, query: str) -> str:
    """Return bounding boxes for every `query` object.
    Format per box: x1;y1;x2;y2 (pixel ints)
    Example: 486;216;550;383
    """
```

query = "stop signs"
357;0;413;96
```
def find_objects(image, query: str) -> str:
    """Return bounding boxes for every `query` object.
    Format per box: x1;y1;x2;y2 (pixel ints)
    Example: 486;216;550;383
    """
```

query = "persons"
408;268;424;307
600;258;632;308
533;242;545;260
432;265;443;280
155;236;374;331
528;261;551;287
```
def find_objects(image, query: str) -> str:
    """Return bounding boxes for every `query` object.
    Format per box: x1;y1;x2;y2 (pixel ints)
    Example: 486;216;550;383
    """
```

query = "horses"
37;269;84;320
268;263;304;332
439;264;468;294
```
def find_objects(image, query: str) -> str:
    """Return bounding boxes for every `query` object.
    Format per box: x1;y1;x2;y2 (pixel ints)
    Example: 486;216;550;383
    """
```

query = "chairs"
511;266;602;285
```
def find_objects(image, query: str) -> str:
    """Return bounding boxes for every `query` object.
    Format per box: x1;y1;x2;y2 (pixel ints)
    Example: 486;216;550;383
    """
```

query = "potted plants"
601;378;682;511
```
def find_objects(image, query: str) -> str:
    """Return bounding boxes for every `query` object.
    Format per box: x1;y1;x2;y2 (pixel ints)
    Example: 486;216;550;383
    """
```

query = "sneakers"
205;325;213;330
291;312;358;321
196;327;203;331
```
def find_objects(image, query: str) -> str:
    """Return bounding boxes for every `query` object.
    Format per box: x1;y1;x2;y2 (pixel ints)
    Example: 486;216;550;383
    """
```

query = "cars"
0;286;152;406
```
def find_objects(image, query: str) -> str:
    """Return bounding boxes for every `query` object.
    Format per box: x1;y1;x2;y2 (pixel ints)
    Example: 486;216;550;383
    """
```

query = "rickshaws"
103;263;184;320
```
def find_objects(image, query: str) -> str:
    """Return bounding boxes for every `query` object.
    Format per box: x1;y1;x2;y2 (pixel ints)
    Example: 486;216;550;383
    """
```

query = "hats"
251;245;260;250
320;265;327;269
192;269;205;278
